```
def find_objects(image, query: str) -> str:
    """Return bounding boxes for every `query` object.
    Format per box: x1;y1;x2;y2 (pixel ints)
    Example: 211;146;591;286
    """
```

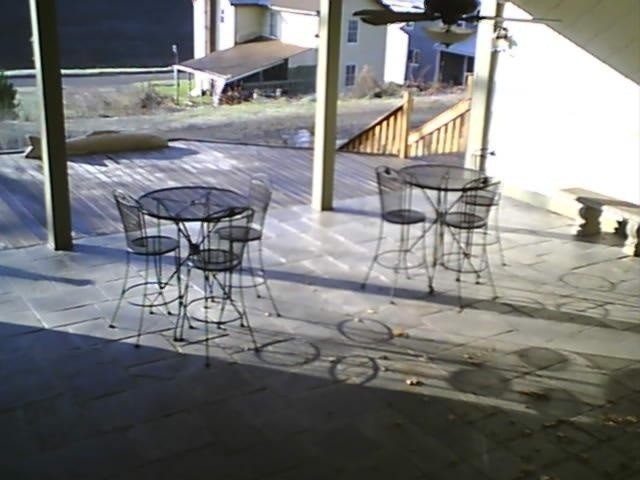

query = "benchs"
559;186;640;257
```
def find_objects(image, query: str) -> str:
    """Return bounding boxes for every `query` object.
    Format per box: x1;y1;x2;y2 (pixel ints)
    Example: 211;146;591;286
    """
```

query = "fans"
351;0;564;51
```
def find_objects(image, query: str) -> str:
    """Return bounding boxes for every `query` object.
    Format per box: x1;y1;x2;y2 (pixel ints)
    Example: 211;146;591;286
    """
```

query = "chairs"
359;163;509;315
107;175;283;370
